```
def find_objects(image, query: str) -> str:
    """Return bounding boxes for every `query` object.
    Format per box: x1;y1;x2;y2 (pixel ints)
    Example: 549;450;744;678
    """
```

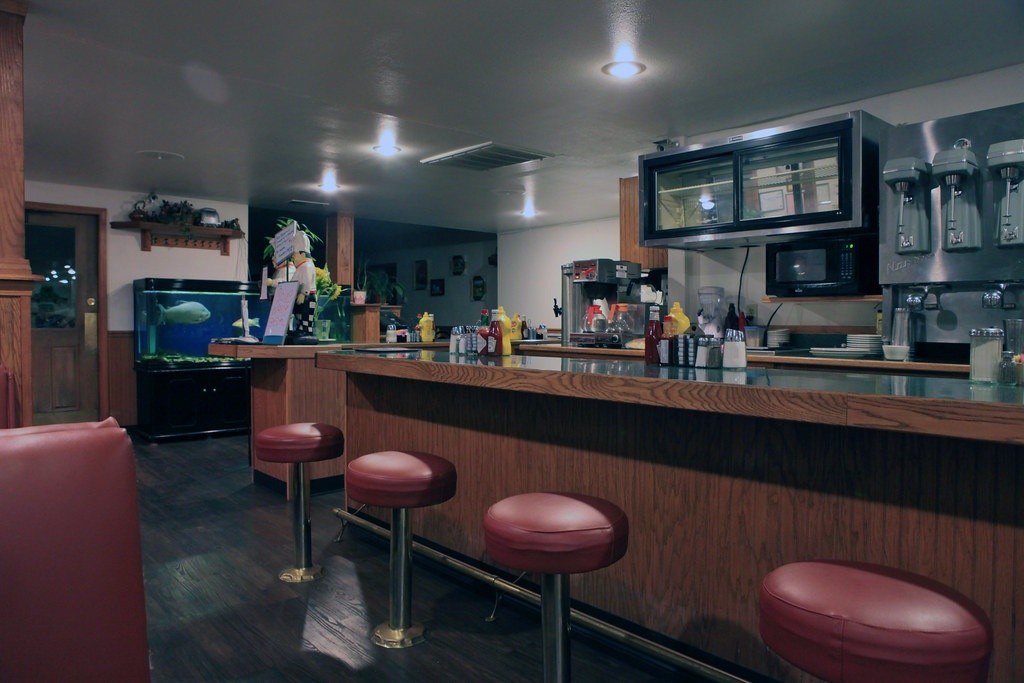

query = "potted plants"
352;261;410;318
159;199;195;225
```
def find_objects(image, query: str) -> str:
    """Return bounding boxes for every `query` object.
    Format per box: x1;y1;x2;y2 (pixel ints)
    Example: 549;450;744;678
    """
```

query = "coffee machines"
560;258;642;349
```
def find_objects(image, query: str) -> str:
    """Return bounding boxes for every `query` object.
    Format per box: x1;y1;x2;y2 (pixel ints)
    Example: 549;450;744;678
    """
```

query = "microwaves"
766;234;877;296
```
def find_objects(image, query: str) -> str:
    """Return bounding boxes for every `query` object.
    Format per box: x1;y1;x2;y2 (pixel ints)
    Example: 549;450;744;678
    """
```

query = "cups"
1003;318;1024;355
744;326;765;347
767;328;790;347
316;320;332;339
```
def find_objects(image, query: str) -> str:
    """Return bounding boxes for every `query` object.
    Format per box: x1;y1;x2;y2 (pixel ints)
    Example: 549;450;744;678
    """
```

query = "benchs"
0;365;149;683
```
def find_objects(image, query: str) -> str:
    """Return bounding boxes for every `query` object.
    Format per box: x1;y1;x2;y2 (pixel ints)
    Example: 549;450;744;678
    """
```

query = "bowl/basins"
883;344;910;361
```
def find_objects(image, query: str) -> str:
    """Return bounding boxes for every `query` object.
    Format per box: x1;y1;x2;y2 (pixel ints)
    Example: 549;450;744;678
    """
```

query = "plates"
810;334;882;356
319;338;336;343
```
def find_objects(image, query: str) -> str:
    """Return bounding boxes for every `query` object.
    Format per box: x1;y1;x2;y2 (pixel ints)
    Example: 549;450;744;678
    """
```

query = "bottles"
644;302;746;369
997;350;1017;386
386;318;397;342
891;307;915;356
414;312;437;342
968;327;1004;384
449;306;547;356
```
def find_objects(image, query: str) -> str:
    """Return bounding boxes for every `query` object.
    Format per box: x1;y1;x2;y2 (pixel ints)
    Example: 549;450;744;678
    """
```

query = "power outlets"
746;303;759;326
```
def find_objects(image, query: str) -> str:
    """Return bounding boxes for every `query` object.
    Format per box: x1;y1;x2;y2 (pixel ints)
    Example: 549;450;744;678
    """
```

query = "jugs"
580;305;606;331
607;303;635;333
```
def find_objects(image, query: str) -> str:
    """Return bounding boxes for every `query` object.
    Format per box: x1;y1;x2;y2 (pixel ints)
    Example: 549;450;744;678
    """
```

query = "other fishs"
231;317;261;329
155;301;211;327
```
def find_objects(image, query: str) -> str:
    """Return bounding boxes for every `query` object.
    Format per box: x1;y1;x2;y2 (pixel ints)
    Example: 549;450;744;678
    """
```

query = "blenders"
697;286;725;338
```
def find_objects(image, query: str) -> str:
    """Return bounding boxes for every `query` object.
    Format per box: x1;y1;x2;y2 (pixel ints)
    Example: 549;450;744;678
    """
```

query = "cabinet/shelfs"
620;110;887;271
138;367;250;444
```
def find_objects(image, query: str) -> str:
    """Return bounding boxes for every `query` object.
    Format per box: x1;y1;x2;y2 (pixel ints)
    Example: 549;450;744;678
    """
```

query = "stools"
758;561;993;683
254;423;344;583
485;492;629;683
345;451;457;649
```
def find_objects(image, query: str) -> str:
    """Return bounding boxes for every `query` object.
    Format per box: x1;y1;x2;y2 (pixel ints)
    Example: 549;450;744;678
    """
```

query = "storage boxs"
133;277;351;371
